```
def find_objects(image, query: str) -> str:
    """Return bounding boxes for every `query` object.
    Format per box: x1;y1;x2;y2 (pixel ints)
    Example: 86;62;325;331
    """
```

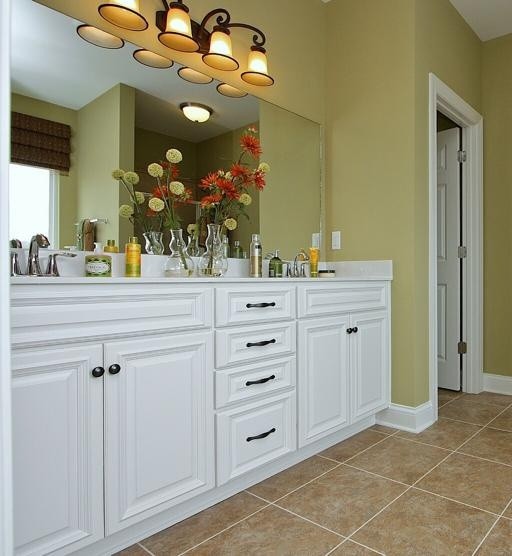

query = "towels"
76;218;95;252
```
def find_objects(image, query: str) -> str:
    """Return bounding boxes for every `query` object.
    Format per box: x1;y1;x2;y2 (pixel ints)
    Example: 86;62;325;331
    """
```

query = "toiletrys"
223;235;231;258
250;234;262;278
318;270;335;278
308;247;320;278
83;242;112;278
268;249;283;278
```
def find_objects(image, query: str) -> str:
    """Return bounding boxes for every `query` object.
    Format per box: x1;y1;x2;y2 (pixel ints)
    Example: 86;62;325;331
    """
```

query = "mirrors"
6;2;325;280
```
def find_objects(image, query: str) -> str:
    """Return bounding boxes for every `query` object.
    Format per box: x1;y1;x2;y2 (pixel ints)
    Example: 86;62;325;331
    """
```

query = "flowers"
111;163;192;253
185;204;227;232
196;122;271;271
145;147;192;268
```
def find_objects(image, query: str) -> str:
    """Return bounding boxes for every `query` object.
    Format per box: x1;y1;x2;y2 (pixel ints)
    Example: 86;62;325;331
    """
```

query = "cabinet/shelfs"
11;276;217;555
298;279;391;461
216;280;298;502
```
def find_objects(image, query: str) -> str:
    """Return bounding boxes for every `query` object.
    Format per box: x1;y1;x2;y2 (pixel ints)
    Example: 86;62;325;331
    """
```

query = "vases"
141;232;165;255
166;230;195;277
200;224;230;277
183;234;207;256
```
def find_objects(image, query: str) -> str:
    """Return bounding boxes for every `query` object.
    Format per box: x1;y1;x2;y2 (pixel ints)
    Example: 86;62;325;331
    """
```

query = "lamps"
95;0;276;89
179;102;213;122
76;21;248;98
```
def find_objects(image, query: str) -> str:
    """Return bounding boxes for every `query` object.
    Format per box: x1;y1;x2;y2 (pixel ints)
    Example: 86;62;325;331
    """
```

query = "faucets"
292;252;309;277
26;234;50;276
266;253;274;258
